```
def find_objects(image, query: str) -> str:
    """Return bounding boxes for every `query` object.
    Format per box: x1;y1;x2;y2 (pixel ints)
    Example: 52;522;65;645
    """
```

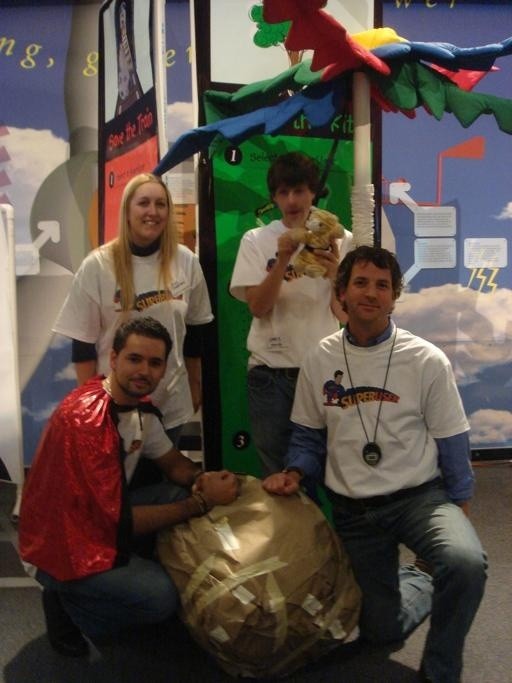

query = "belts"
329;476;442;508
256;364;300;378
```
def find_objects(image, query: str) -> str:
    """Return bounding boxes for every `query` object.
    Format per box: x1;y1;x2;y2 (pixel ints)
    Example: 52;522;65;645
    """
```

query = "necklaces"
104;375;121;405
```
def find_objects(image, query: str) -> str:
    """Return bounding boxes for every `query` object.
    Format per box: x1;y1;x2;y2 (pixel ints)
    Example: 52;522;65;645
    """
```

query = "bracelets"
191;491;210;513
187;469;204;492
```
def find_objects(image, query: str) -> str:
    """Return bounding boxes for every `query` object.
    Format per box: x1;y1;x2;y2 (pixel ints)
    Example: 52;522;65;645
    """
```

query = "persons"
19;316;241;658
227;150;352;474
52;171;217;445
262;243;490;680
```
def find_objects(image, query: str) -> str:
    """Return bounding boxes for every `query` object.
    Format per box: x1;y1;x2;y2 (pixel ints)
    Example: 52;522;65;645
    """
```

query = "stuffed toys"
283;205;346;279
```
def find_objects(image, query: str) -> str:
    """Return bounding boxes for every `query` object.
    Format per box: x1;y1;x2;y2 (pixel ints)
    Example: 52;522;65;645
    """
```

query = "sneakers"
41;587;91;657
